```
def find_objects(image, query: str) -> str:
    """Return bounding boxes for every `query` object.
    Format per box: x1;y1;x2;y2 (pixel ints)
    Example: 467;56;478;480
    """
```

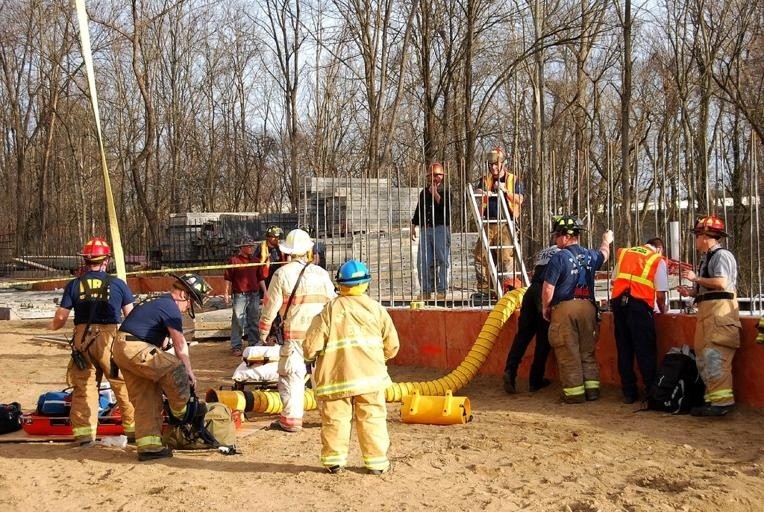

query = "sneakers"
416;293;432;300
560;392;586;403
690;403;710;417
138;448;173;461
434;292;446;300
702;402;736;416
586;390;600;401
528;378;550;393
503;371;517;394
270;420;293;432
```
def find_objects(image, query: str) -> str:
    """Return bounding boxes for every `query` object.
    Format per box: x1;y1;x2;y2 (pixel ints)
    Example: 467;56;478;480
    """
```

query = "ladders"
466;182;531;300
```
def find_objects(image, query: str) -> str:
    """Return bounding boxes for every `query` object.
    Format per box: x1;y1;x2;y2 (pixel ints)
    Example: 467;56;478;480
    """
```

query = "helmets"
693;214;729;240
278;229;316;256
551;215;582;236
265;224;284;237
486;149;507;165
77;237;112;260
425;163;447;178
333;258;373;285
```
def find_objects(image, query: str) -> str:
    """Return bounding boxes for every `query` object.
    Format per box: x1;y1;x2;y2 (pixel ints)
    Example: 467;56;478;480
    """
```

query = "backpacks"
634;346;700;415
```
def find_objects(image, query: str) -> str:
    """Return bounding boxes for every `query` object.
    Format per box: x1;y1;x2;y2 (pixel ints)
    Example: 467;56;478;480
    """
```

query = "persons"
502;236;562;395
111;273;205;461
409;163;454;303
258;229;338;434
48;238;134;448
609;238;670;404
254;225;291;342
542;215;614;404
470;148;525;303
223;240;266;357
302;260;401;476
677;215;742;419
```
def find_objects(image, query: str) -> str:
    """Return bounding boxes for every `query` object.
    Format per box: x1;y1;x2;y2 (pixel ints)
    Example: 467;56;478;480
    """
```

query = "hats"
168;271;211;306
234;236;257;247
